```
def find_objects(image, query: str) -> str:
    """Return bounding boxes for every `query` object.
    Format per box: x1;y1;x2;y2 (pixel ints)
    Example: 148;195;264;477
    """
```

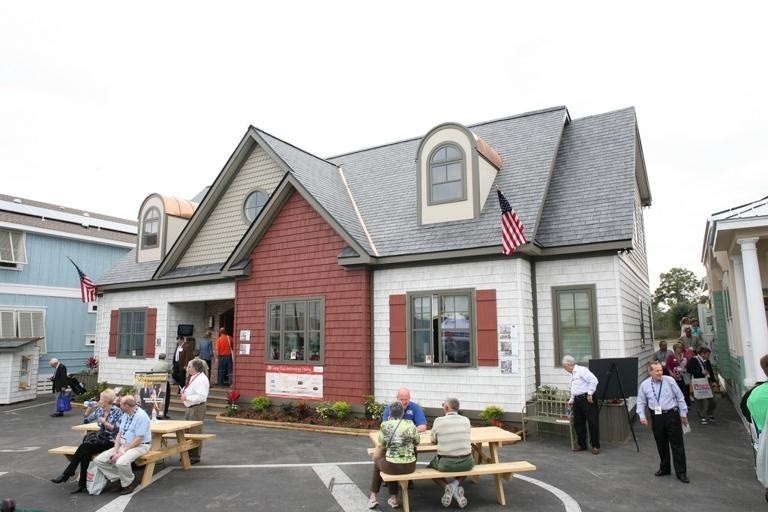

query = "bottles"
152;408;157;422
83;400;98;407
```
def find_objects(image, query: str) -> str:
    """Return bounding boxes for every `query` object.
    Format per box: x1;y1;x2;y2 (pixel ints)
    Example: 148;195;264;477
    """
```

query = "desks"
368;422;523;512
69;413;205;486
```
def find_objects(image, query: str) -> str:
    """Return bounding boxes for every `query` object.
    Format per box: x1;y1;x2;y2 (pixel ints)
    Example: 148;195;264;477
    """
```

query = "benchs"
48;442;165;485
164;430;215;471
364;442;504;462
376;459;538;510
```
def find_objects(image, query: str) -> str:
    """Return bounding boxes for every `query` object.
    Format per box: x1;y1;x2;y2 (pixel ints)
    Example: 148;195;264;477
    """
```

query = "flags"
495;188;528;257
73;263;98;303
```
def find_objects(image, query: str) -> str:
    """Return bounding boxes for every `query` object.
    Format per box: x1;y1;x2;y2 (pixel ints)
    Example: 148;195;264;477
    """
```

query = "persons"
561;354;602;456
740;354;768;500
426;396;475;508
196;330;214;388
172;335;193;394
366;401;420;508
91;395;153;495
48;358;69;418
150;352;174;419
214;328;236;389
140;381;164;420
653;316;723;425
178;359;210;464
50;388;124;495
185;349;209;411
382;387;428;489
635;359;691;484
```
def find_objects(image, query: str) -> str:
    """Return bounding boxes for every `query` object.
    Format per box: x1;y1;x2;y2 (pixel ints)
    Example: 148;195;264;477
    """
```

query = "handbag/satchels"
691;378;713;400
231;348;235;362
57;388;71;411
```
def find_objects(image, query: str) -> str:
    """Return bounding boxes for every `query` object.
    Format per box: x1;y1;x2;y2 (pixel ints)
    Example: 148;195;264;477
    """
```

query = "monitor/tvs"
178;325;193;336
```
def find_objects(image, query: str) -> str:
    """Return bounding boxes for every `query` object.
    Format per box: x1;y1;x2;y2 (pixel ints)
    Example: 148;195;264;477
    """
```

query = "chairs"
520;388;578;451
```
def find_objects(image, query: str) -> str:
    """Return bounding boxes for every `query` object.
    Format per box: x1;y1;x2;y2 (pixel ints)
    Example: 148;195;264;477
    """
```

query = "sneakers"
441;484;468;508
382;482;388;487
368;500;379;509
700;416;715;425
387;498;399;508
408;480;415;489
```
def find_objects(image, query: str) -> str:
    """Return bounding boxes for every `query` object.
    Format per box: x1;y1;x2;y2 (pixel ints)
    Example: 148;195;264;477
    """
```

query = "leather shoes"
675;471;688;483
50;412;63;417
655;468;671;475
592;447;599;455
572;445;587;451
180;456;200;465
110;479;138;494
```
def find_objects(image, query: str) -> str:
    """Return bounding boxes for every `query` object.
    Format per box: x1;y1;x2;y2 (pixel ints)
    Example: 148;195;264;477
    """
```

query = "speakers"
208;315;214;328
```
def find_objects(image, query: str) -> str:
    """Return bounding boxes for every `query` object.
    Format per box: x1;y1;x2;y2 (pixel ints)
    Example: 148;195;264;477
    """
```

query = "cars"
411;312;470;365
268;338;319;361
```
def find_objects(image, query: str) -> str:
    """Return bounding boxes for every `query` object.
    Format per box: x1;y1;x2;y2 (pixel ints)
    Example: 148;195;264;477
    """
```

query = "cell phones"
46;377;52;381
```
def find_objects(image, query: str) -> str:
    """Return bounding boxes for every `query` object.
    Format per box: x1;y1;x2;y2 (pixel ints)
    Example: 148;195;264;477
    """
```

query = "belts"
573;391;592;398
438;453;471;459
650;405;679;414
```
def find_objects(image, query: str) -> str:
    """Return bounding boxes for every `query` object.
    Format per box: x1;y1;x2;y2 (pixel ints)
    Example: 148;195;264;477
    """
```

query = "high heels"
51;473;70;483
70;485;86;494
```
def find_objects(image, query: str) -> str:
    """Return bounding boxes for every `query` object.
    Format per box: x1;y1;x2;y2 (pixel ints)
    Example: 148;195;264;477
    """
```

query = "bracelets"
587;394;592;396
